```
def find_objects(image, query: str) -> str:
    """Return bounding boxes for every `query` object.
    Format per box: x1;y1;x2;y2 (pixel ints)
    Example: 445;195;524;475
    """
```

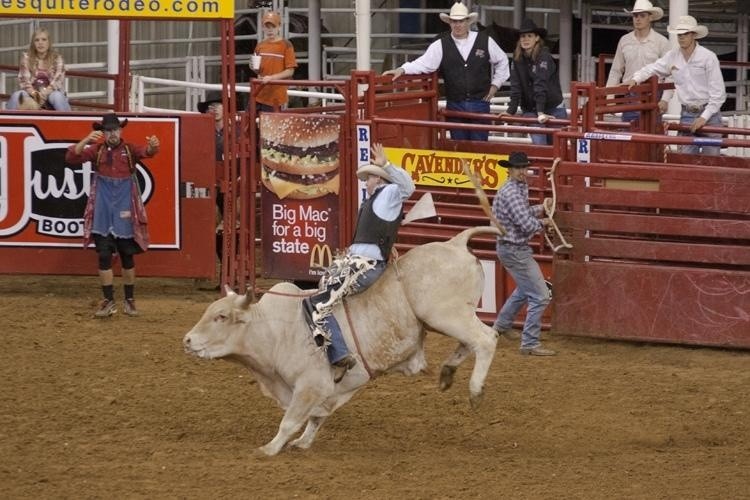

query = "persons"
65;113;160;318
497;17;568;177
246;12;297;162
7;26;71;112
617;15;727;155
197;91;249;290
605;0;674;132
381;1;511;140
492;151;556;356
304;142;416;382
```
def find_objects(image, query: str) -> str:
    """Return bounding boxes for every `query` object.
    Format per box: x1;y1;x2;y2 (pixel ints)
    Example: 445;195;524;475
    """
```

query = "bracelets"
382;162;390;169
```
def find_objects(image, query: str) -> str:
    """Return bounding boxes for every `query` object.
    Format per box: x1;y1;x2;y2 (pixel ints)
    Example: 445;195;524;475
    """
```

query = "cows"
181;157;508;461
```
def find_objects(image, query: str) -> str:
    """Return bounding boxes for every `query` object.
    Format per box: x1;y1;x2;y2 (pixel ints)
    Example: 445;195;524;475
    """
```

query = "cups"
249;55;262;70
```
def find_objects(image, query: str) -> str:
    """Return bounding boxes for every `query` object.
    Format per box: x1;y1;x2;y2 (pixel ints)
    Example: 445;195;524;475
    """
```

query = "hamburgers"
261;114;340;200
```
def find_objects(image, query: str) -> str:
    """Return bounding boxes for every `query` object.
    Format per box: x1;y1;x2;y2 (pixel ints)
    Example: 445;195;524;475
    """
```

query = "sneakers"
123;299;137;316
521;347;555;356
334;356;356;383
96;298;117;317
495;329;520;340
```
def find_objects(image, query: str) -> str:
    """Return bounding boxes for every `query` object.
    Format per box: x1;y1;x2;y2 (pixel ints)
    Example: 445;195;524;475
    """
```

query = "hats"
263;12;280;26
667;16;709;39
518;19;547;38
498;151;531;168
439;2;479;27
92;112;128;131
197;92;223;113
355;164;392;183
624;0;664;22
